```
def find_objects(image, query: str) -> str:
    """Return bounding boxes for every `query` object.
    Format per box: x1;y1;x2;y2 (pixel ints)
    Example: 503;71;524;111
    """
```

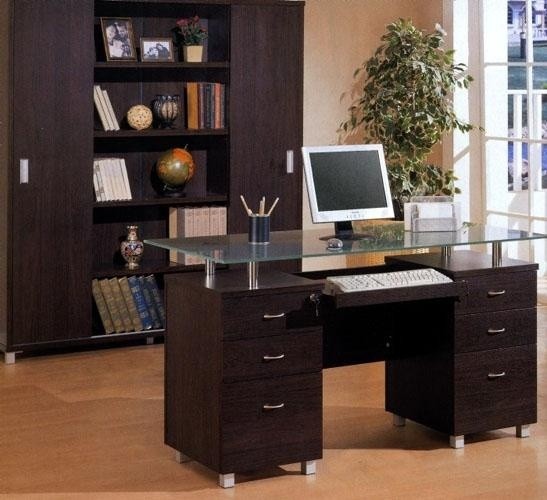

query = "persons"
145;48;153;60
155;43;169;59
147;47;163;59
107;38;124;58
114;21;132;57
105;22;117;45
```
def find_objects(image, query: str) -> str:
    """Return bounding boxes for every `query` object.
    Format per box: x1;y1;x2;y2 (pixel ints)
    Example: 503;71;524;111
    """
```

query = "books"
90;273;166;335
166;205;228;265
92;86;109;132
95;85;114;132
91;158;133;203
184;80;225;131
102;89;120;132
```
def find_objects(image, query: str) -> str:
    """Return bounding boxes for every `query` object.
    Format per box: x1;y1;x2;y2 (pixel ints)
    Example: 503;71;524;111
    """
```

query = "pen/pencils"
239;195;279;217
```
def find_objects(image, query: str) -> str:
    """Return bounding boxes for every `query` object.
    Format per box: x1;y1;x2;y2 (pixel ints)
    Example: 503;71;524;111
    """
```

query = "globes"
155;148;195;196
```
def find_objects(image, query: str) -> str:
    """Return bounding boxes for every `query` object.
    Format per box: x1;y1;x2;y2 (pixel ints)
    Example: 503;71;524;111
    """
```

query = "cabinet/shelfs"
163;271;326;497
93;3;233;345
383;251;539;451
233;1;302;233
3;3;94;362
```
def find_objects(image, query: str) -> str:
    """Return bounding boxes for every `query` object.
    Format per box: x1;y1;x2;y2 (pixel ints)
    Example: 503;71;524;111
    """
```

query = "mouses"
328;238;343;248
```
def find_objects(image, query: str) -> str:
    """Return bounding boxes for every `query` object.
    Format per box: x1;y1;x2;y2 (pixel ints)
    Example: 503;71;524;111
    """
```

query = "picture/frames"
139;37;175;62
101;17;135;61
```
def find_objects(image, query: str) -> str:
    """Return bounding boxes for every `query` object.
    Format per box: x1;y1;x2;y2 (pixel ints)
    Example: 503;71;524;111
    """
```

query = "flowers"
178;16;206;45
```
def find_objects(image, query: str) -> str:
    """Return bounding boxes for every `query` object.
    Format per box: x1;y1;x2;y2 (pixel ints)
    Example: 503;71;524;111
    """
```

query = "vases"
183;46;203;63
120;225;144;269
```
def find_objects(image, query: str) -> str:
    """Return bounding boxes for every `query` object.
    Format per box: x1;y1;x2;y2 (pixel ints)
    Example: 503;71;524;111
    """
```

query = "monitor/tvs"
301;143;395;241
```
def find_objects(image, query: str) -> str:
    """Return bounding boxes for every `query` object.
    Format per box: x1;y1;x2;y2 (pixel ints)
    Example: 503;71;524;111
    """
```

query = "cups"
247;213;271;246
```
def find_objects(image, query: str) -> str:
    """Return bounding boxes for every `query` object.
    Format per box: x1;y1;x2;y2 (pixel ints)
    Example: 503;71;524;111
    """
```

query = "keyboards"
325;268;453;294
